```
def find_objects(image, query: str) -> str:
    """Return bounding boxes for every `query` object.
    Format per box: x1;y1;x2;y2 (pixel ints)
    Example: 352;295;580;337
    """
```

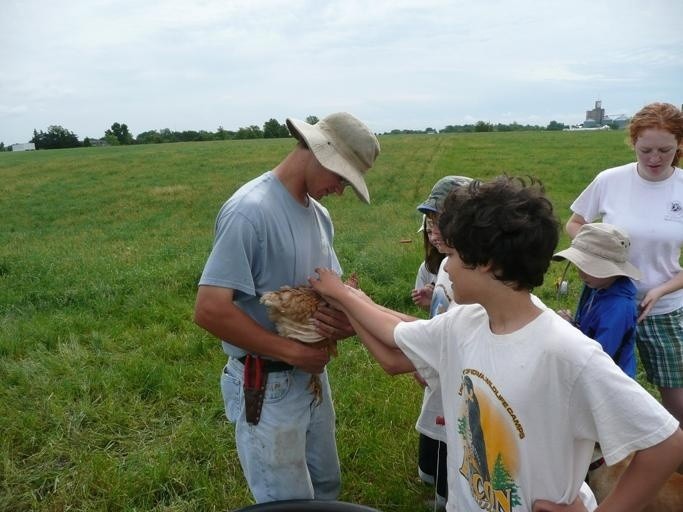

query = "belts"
236;353;296;374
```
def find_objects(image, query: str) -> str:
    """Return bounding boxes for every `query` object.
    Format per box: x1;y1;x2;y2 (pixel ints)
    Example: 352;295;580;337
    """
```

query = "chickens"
258;272;359;410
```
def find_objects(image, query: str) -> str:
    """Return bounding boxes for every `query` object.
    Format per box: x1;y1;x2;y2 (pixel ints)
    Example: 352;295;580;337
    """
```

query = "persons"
310;176;683;512
567;103;682;429
194;109;382;505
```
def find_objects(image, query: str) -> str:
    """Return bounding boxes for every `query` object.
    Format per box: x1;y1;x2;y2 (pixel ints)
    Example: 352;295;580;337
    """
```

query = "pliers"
242;352;260;396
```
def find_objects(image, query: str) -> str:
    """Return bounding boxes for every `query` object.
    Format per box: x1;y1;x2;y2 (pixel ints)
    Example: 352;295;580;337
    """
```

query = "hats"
415;175;479;219
285;109;377;205
551;222;642;282
